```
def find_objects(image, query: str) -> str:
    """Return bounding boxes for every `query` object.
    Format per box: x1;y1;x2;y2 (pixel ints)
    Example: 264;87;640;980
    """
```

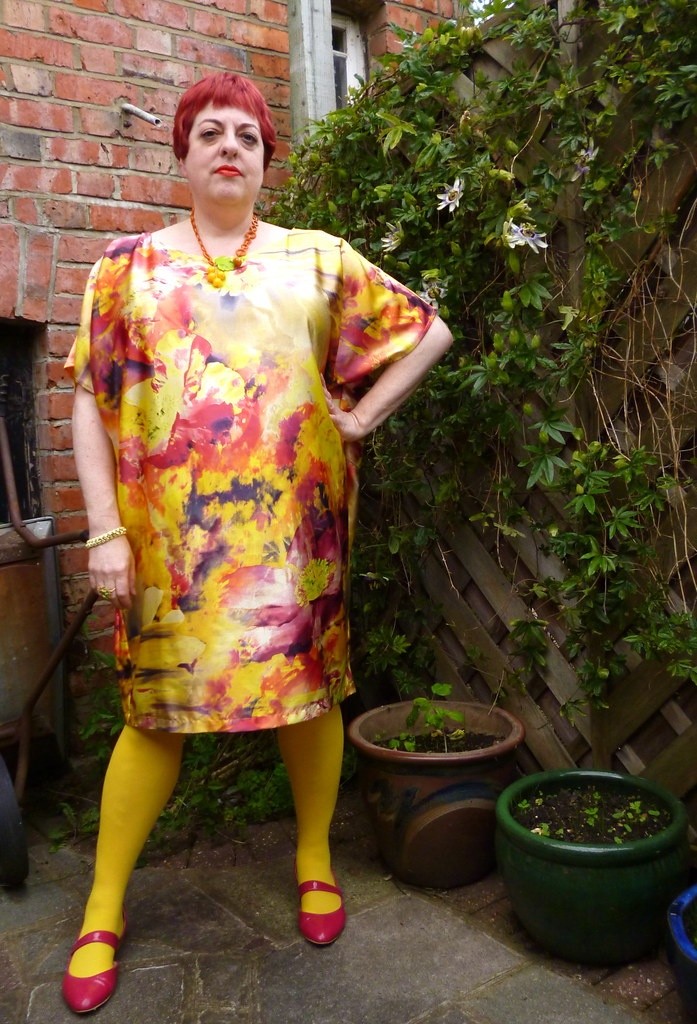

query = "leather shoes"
62;903;128;1012
294;856;346;945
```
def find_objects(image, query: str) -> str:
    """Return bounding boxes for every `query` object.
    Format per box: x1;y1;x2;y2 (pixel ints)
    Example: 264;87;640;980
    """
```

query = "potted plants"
348;683;525;888
495;768;690;964
666;884;697;1020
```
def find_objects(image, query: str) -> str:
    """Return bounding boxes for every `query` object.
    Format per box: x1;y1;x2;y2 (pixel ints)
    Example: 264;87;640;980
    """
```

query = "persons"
63;73;454;1012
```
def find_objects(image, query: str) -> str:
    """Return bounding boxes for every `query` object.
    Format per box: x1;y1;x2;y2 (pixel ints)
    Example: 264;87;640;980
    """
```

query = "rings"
97;585;116;599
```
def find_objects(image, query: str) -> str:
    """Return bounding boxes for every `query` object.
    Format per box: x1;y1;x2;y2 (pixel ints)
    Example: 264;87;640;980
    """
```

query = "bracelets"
87;527;128;550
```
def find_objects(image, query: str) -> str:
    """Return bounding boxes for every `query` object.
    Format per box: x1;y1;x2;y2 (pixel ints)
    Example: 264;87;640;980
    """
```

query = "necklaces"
189;206;258;271
